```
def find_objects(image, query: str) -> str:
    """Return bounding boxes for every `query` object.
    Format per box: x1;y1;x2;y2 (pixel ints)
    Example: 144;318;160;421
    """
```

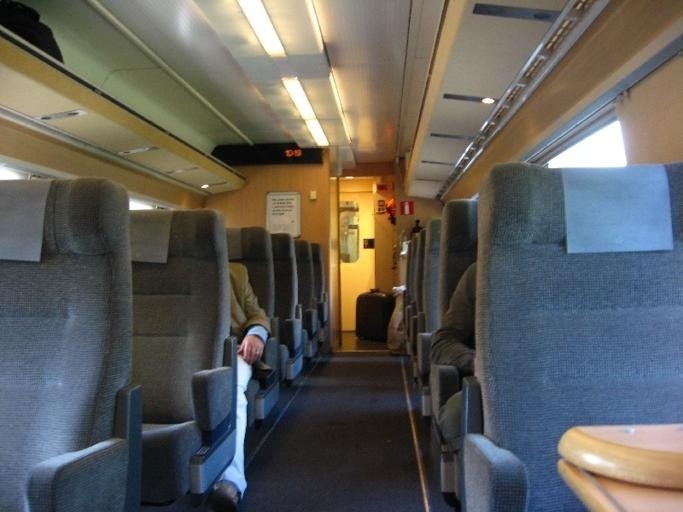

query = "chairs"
0;177;327;511
398;164;683;512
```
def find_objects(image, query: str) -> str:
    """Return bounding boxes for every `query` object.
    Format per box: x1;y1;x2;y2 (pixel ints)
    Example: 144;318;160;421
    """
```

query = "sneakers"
212;480;241;509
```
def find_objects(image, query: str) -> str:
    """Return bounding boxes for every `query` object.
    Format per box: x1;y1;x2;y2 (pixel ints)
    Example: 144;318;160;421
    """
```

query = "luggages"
356;288;394;341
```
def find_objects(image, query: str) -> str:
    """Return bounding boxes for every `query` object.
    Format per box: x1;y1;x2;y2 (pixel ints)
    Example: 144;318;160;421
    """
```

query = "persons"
428;259;478;508
388;282;407;355
208;260;272;510
